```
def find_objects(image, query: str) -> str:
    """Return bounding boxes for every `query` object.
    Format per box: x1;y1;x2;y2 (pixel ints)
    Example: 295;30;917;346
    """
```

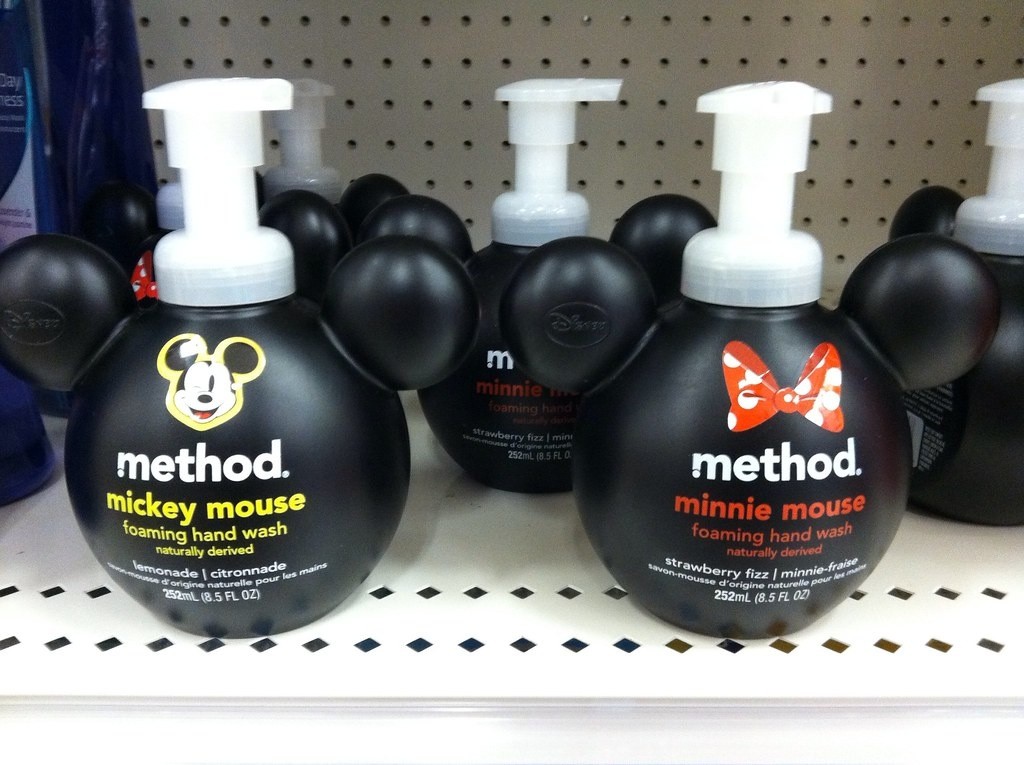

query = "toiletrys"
0;1;1021;641
